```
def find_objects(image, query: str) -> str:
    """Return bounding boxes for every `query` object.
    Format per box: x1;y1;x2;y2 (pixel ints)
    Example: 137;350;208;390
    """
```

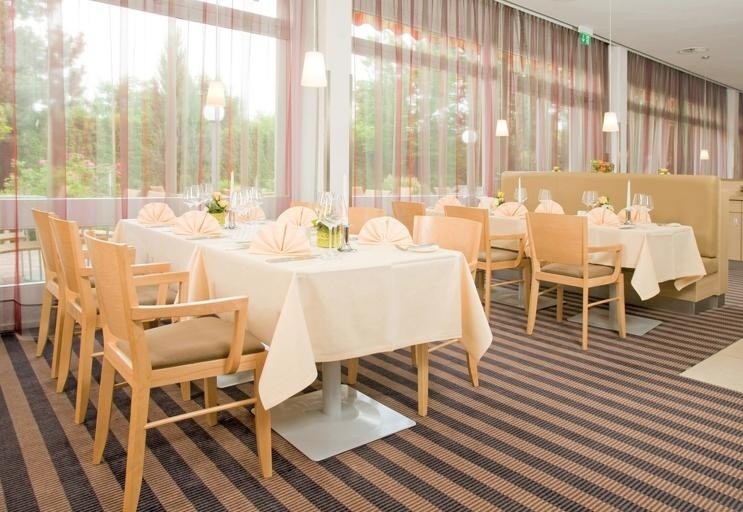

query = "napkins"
355;217;412;247
477;199;498;210
494;202;528;217
137;202;176;225
176;210;225;239
588;206;619;227
534;200;563;214
247;223;313;257
434;198;464;215
276;206;318;227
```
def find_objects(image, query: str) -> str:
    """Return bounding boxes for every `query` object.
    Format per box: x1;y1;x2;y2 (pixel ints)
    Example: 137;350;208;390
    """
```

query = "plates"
397;242;439;252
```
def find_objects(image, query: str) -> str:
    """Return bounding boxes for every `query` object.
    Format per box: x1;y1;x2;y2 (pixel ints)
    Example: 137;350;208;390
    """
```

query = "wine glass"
318;191;357;263
182;182;212;212
632;193;654;229
514;187;528;204
582;190;599;213
538;188;552;213
231;186;263;233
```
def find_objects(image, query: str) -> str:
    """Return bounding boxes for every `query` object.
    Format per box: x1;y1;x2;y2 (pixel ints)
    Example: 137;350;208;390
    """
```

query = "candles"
627;180;631;207
518;178;522;201
230;171;235;198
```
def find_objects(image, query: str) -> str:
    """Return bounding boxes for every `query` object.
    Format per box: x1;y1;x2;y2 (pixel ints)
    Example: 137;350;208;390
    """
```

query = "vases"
316;226;343;248
207;212;228;227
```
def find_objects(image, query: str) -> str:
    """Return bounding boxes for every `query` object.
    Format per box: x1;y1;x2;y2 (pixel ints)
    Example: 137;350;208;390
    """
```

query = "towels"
618;206;651;225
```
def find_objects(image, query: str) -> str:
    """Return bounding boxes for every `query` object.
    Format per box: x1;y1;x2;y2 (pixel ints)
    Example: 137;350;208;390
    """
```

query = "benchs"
501;172;727;316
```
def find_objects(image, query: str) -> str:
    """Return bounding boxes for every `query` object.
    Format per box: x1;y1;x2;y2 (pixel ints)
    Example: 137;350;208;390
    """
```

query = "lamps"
496;2;509;136
699;55;709;160
207;1;227;109
601;0;619;133
301;0;328;88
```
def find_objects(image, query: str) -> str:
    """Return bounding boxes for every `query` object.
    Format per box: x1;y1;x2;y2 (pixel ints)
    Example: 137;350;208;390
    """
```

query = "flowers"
593;194;611;208
496;192;506;206
591;160;614;171
311;204;349;234
201;192;231;213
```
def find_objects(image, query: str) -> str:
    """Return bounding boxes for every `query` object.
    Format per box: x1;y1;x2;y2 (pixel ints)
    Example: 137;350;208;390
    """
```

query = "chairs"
346;206;383;239
443;204;532;319
84;229;278;511
291;202;322;226
32;209;101;378
392;201;426;241
523;210;624;351
345;215;483;417
50;215;193;425
